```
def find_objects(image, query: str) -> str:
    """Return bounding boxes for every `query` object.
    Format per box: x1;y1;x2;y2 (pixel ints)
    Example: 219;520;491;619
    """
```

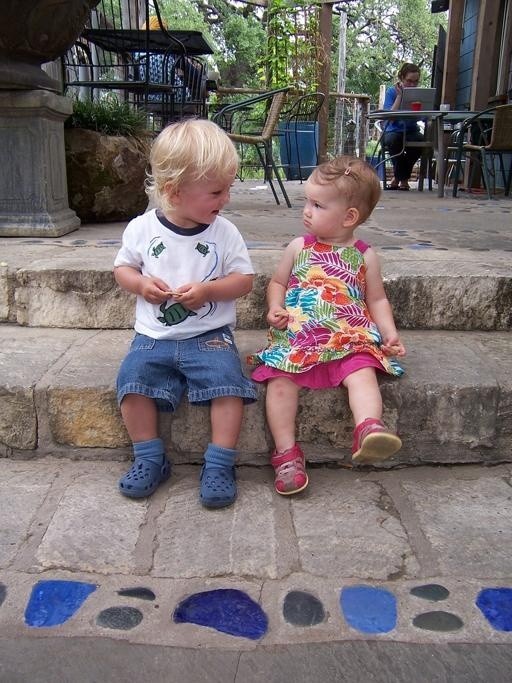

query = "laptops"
399;87;437;110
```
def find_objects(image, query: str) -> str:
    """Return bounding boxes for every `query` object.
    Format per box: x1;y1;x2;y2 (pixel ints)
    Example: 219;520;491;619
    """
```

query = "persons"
383;63;426;190
132;16;210;129
247;155;407;496
113;116;257;509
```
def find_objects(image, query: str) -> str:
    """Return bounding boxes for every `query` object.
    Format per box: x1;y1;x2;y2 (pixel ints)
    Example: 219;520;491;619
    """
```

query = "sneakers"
273;442;308;495
351;418;402;466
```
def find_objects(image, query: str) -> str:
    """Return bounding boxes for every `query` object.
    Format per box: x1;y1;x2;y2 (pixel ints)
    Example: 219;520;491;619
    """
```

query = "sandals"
199;457;237;508
118;453;170;497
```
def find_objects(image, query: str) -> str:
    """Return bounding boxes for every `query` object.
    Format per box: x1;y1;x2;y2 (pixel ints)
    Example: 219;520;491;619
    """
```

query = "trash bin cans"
366;156;384;181
279;121;320;180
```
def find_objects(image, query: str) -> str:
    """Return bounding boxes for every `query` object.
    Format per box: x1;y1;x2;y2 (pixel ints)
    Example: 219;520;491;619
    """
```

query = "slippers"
386;183;410;190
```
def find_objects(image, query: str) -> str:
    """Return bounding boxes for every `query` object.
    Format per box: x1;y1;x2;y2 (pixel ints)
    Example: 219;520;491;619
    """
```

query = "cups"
440;104;451;111
411;102;422;111
